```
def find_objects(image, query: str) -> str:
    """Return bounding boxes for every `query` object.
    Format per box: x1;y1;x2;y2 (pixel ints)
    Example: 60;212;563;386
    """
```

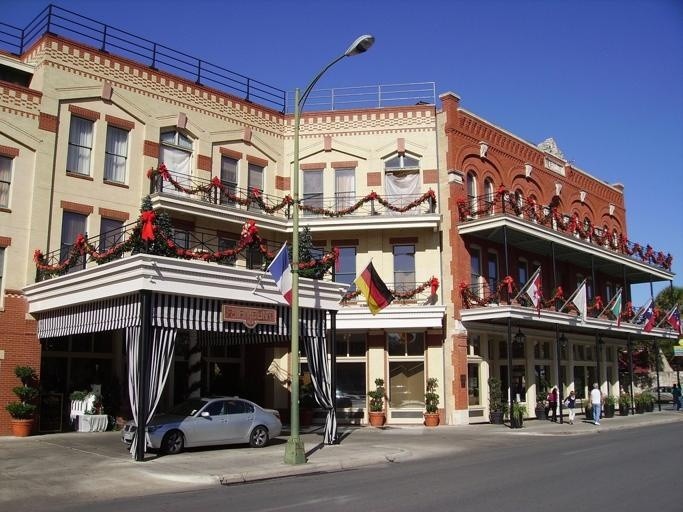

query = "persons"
564;391;576;424
547;384;559;422
590;382;601;424
671;383;683;412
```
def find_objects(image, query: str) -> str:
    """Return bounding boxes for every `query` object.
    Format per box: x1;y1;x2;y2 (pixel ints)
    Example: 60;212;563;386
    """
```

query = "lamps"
558;334;567;348
511;327;525;345
598;337;604;351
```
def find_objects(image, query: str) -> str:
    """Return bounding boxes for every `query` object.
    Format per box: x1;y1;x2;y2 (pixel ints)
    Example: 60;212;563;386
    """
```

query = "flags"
611;294;623;328
268;245;293;308
526;272;543;318
572;284;588;323
642;301;654;332
355;263;395;317
668;307;682;335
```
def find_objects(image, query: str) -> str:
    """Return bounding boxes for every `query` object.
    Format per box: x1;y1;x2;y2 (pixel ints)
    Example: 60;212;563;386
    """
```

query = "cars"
120;393;283;454
395;385;412;394
300;389;352;407
642;386;677;404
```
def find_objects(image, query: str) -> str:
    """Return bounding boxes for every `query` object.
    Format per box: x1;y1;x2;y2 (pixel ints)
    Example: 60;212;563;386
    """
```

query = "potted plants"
487;379;507;429
531;376;551;418
299;383;316;427
417;377;443;427
510;396;524;430
584;390;656;422
366;375;390;429
4;358;42;437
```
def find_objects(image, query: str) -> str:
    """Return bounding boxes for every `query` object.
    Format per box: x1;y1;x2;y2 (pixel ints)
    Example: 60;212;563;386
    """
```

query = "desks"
75;414;108;433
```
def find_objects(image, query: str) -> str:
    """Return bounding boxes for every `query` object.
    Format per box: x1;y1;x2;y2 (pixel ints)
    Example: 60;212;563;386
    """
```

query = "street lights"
283;34;375;465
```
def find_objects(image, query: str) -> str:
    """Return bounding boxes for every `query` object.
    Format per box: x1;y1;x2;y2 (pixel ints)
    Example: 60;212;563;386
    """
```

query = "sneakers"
568;419;573;425
595;422;600;425
